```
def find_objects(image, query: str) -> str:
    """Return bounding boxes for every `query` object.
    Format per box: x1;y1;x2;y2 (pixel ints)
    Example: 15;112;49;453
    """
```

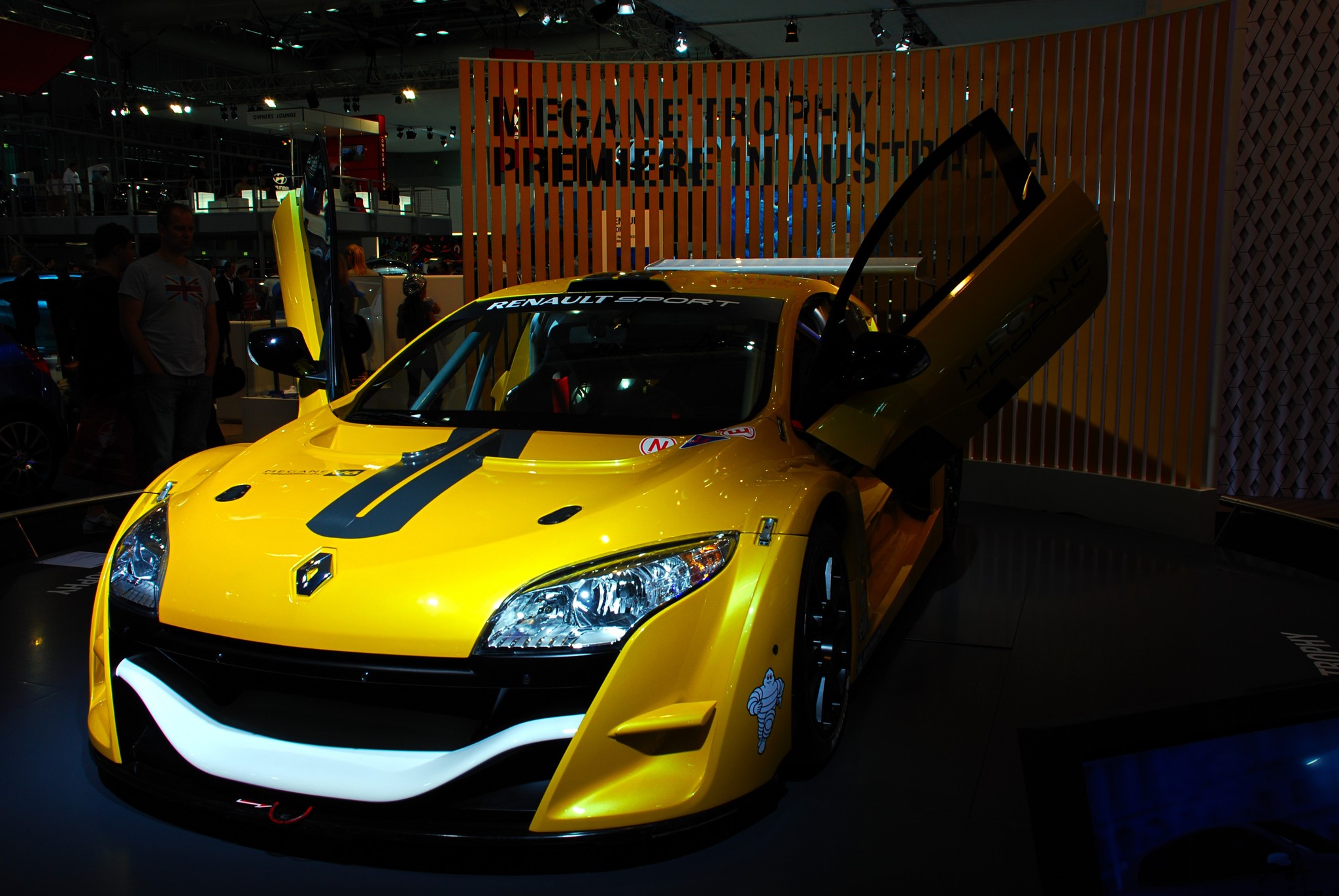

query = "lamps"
395;124;456;147
617;0;635;15
220;104;266;121
343;94;360;113
709;39;722;57
541;8;568;26
869;8;883;38
893;22;914;55
393;91;412;105
784;15;801;43
675;30;688;53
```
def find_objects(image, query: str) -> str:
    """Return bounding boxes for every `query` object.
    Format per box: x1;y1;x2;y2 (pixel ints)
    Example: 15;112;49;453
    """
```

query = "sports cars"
87;106;1108;841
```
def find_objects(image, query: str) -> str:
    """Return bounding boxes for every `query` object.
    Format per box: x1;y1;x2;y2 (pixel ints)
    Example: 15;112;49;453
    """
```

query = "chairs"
248;325;326;396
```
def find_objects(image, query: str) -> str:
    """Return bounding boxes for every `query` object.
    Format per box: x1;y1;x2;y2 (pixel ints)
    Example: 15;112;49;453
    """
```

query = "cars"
0;272;82;339
364;258;410;276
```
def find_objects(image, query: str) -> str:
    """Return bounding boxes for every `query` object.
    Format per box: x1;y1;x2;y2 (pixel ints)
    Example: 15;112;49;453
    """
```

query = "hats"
349;279;364;297
402;274;426;295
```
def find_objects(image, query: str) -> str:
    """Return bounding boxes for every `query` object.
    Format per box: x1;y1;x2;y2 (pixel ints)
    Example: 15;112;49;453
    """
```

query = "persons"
0;152;596;398
396;273;447;409
119;201;221;478
